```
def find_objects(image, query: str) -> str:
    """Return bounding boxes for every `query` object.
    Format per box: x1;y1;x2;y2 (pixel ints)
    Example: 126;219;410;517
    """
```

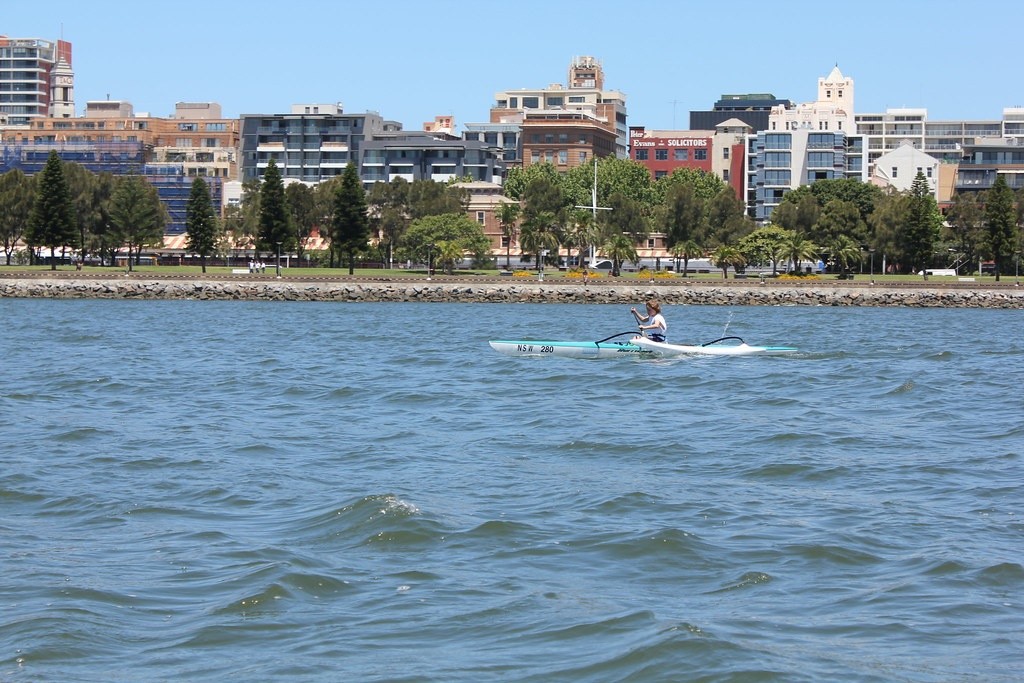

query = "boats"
488;324;799;356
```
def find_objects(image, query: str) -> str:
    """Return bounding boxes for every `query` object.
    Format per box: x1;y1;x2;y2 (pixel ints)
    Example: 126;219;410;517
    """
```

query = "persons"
261;260;266;274
255;260;261;274
248;260;254;273
631;299;667;344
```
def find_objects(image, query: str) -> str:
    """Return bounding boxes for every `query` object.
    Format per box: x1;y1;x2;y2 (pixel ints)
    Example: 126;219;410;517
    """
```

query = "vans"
917;268;956;277
